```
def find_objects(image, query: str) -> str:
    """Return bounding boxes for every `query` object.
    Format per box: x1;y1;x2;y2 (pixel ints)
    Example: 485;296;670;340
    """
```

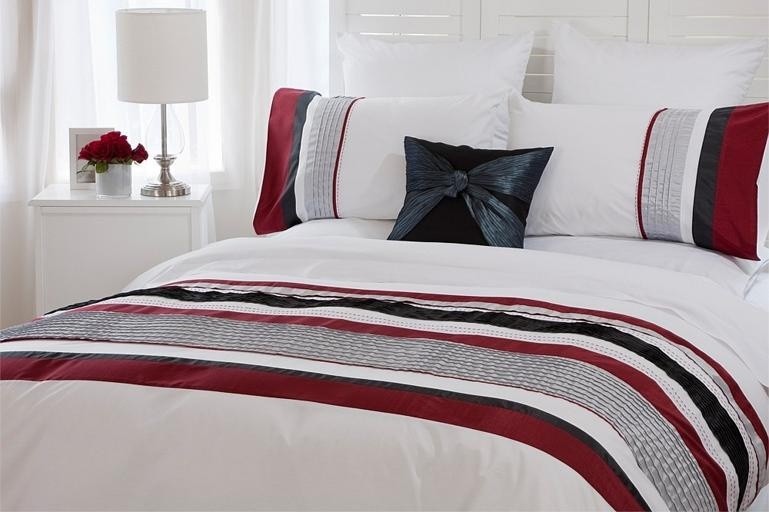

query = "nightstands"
28;181;213;318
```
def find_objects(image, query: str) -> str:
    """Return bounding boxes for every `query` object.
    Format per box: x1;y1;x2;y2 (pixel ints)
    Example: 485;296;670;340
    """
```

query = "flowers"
77;131;148;175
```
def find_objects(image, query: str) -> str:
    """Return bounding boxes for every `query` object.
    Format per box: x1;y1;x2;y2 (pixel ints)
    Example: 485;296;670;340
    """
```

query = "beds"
0;0;768;511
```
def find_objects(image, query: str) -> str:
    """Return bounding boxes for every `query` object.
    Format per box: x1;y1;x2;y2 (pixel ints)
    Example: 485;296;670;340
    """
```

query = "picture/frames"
68;128;115;190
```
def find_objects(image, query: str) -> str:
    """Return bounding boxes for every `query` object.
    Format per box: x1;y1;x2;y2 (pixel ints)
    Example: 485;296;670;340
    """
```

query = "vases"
94;162;133;195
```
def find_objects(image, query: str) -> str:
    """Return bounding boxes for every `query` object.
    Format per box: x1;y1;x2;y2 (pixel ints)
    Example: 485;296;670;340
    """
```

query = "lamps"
117;10;207;197
